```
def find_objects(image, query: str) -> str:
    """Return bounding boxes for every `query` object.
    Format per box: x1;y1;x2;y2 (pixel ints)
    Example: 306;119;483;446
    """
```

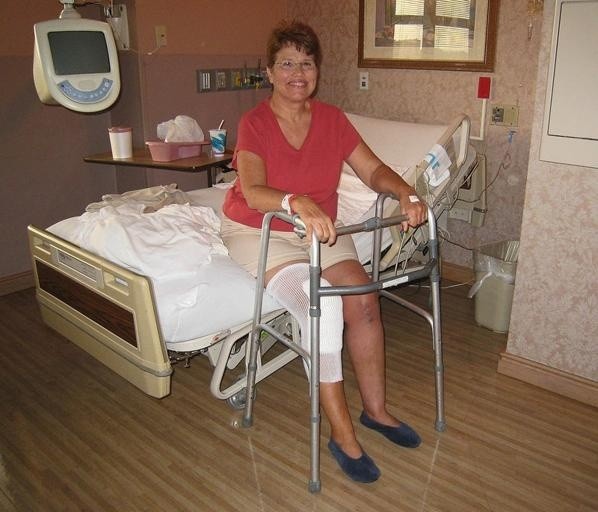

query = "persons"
219;19;429;482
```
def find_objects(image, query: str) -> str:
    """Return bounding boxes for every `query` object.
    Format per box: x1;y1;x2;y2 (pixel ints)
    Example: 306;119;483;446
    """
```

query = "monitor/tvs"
32;18;121;112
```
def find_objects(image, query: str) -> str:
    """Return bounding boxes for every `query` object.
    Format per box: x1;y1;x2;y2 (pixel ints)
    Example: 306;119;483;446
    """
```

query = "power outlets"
155;25;167;48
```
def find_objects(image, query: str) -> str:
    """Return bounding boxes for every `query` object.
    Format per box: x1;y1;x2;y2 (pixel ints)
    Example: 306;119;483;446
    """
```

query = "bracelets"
281;193;298;215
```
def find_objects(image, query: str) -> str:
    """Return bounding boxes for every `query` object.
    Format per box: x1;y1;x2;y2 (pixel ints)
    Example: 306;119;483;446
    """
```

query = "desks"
83;147;234;188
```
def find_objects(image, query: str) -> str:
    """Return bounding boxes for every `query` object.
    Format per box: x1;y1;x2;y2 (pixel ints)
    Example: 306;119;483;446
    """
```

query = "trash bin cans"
474;237;520;334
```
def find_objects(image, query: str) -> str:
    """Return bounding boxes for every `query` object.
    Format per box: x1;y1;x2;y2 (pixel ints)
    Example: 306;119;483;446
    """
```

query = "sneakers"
360;410;421;448
328;439;380;483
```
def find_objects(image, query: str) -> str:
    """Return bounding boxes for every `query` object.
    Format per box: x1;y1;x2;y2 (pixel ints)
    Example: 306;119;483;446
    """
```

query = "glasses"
275;60;315;70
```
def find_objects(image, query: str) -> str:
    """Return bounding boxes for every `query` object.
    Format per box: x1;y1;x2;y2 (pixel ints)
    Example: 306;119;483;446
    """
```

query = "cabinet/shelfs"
538;0;598;169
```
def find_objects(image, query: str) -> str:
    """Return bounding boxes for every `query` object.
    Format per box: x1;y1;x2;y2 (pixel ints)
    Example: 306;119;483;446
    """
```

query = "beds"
27;114;488;411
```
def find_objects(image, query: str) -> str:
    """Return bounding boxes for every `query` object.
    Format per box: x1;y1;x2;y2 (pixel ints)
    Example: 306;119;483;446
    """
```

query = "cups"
208;129;227;154
